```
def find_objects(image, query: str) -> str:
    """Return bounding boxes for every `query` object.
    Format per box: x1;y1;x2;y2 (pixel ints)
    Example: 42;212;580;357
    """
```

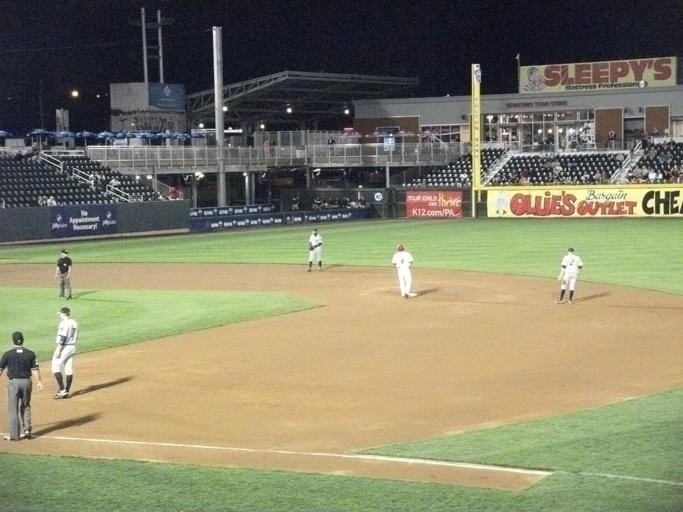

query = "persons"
64;163;74;182
88;170;120;194
14;151;24;163
421;126;431;142
53;248;72;300
262;137;270;147
326;136;335;145
509;126;683;186
45;195;57;207
48;306;78;399
302;228;323;272
36;196;47;207
390;243;419;298
311;191;368;215
0;330;43;442
553;247;583;305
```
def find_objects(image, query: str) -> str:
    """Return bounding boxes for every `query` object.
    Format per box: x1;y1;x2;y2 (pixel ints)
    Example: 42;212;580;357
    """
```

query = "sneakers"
25;430;31;439
4;435;20;440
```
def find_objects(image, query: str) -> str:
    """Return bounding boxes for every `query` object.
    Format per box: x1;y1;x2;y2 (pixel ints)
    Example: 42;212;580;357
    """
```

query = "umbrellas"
114;129;137;145
74;129;94;147
96;130;115;145
52;130;77;150
30;128;51;150
25;132;37;145
0;129;17;150
140;126;202;146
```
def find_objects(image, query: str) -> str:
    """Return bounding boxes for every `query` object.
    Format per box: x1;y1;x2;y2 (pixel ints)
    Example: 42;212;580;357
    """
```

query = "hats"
57;308;70;314
62;250;68;254
13;332;23;340
